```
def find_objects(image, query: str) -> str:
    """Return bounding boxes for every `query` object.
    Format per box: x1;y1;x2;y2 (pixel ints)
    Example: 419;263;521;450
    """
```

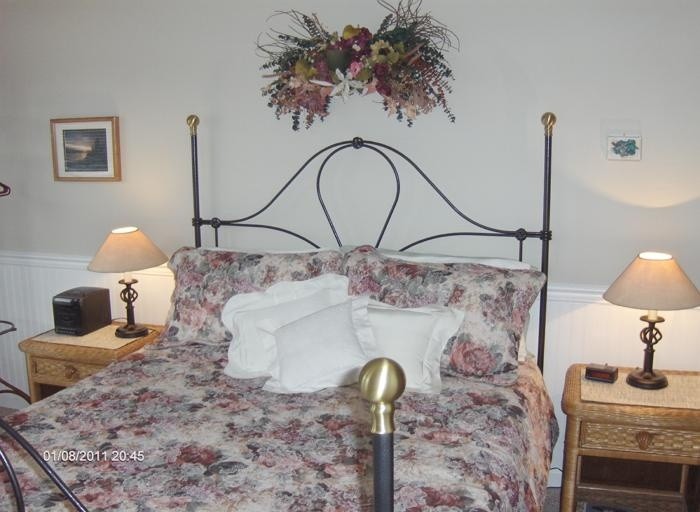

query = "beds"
0;112;559;512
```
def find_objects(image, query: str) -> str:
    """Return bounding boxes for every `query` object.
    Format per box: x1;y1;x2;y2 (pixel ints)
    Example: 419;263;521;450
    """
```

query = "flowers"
252;0;460;132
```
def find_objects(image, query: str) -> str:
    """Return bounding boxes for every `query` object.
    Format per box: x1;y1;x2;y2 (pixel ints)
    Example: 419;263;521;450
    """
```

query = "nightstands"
19;320;165;405
560;362;700;512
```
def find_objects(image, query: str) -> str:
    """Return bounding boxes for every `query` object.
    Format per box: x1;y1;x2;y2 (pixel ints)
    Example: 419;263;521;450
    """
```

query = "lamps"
602;251;700;390
87;226;170;338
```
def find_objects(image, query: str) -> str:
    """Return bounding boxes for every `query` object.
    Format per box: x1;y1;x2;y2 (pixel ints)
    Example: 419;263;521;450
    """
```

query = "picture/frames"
51;116;121;182
